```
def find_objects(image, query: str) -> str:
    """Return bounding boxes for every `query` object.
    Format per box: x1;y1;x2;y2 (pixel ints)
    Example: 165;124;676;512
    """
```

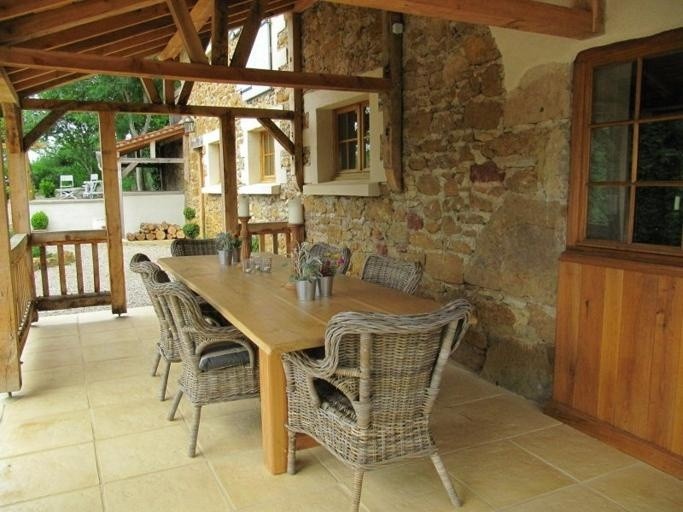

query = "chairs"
303;240;351;276
58;174;98;200
148;267;259;457
130;253;240;400
169;236;234;328
361;254;424;297
278;299;472;511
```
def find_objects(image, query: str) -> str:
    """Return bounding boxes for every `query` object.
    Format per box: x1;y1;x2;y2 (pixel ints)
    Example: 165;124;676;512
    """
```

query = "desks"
157;253;446;476
56;187;82;199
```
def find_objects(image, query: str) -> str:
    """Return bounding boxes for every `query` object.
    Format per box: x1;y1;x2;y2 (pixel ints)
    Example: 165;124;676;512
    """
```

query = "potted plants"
290;245;323;301
316;246;338;296
229;237;241;263
216;236;234;266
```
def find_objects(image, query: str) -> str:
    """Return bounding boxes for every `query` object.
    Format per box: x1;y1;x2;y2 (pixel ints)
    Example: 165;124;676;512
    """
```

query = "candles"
239;198;249;216
288;202;304;225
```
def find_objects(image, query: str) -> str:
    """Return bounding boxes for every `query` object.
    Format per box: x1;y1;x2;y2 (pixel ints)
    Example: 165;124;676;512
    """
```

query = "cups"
240;255;272;273
